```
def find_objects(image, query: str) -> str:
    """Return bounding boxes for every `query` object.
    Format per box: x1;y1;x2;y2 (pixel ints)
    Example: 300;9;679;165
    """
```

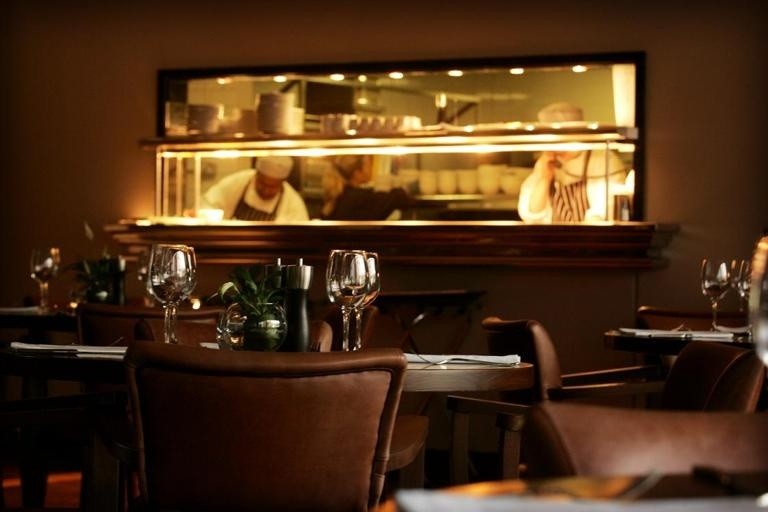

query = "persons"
201;157;311;222
318;155;346;219
331;155;412;220
516;101;628;222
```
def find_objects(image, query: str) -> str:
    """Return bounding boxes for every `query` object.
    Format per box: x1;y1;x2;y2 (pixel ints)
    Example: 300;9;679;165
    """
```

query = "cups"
358;115;412;131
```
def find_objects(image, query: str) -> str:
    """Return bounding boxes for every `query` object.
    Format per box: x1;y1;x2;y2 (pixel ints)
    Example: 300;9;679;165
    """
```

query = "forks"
408;346;520;367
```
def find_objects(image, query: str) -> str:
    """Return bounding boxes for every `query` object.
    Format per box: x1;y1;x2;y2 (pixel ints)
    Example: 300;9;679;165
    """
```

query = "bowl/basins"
399;163;521;197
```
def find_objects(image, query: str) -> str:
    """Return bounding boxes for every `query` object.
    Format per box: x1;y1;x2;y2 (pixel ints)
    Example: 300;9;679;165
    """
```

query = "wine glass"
698;256;754;335
28;244;60;313
146;241;199;346
324;247;382;353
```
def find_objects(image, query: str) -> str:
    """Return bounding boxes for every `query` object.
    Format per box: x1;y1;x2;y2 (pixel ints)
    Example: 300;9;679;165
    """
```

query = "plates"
164;91;306;136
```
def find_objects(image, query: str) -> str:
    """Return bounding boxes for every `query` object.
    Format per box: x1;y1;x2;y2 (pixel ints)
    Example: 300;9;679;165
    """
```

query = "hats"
255;156;294;179
538;103;582;123
